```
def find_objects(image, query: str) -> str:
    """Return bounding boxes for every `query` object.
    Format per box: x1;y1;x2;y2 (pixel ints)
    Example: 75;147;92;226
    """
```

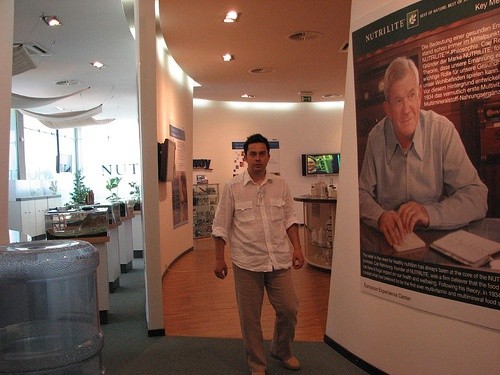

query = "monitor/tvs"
159;138;177;182
304;154;340;176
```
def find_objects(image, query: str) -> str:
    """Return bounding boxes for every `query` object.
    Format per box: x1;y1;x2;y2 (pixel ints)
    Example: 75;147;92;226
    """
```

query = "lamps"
41;14;63;28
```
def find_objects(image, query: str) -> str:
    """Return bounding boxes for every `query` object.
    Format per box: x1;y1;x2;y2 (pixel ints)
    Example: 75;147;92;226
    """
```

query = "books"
391;228;425;252
431;229;500;267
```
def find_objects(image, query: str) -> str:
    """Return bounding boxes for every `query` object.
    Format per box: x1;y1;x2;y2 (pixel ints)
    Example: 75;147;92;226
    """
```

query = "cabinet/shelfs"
192;183;221;239
8;197;62;244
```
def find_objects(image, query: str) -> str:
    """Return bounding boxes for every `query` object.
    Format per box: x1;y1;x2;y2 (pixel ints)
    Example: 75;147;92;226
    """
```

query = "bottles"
310;182;337;198
86;190;94;205
312;215;333;244
0;240;106;375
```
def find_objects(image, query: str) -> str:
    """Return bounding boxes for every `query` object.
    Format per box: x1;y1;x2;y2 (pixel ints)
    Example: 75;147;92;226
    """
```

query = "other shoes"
251;369;266;375
270;348;300;370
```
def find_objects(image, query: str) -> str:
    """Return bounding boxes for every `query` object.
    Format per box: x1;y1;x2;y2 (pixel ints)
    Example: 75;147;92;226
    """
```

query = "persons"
210;132;305;375
357;55;488;247
307;157;324;174
